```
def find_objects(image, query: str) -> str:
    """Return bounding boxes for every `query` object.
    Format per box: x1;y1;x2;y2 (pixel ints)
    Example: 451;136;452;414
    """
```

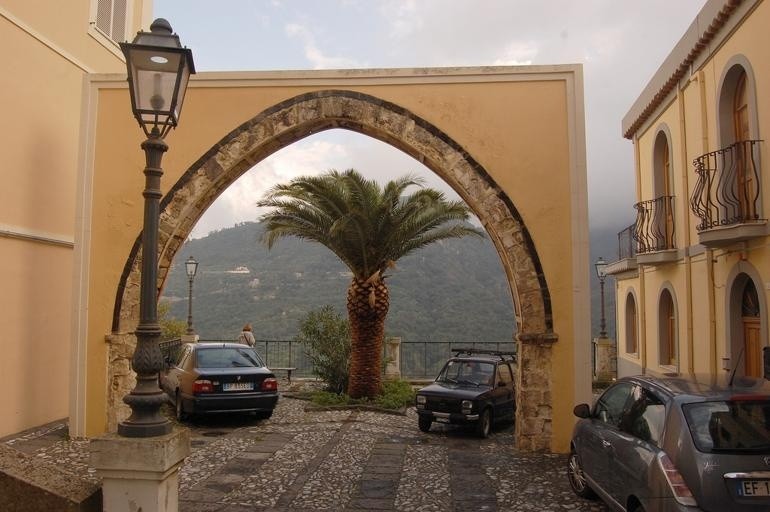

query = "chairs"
470;371;490;385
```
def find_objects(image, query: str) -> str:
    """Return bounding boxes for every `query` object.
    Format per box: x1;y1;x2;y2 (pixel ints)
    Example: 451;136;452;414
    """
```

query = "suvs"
415;347;518;440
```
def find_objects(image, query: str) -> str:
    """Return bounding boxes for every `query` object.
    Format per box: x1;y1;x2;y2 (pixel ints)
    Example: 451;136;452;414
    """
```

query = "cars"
160;343;279;422
568;372;769;512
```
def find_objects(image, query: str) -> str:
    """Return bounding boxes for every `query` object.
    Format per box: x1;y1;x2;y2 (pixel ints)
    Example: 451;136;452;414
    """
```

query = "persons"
238;323;256;360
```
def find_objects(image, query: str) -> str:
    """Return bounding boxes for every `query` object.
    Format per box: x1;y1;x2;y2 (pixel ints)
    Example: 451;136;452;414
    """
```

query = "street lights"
594;256;608;339
118;18;196;438
185;256;199;335
